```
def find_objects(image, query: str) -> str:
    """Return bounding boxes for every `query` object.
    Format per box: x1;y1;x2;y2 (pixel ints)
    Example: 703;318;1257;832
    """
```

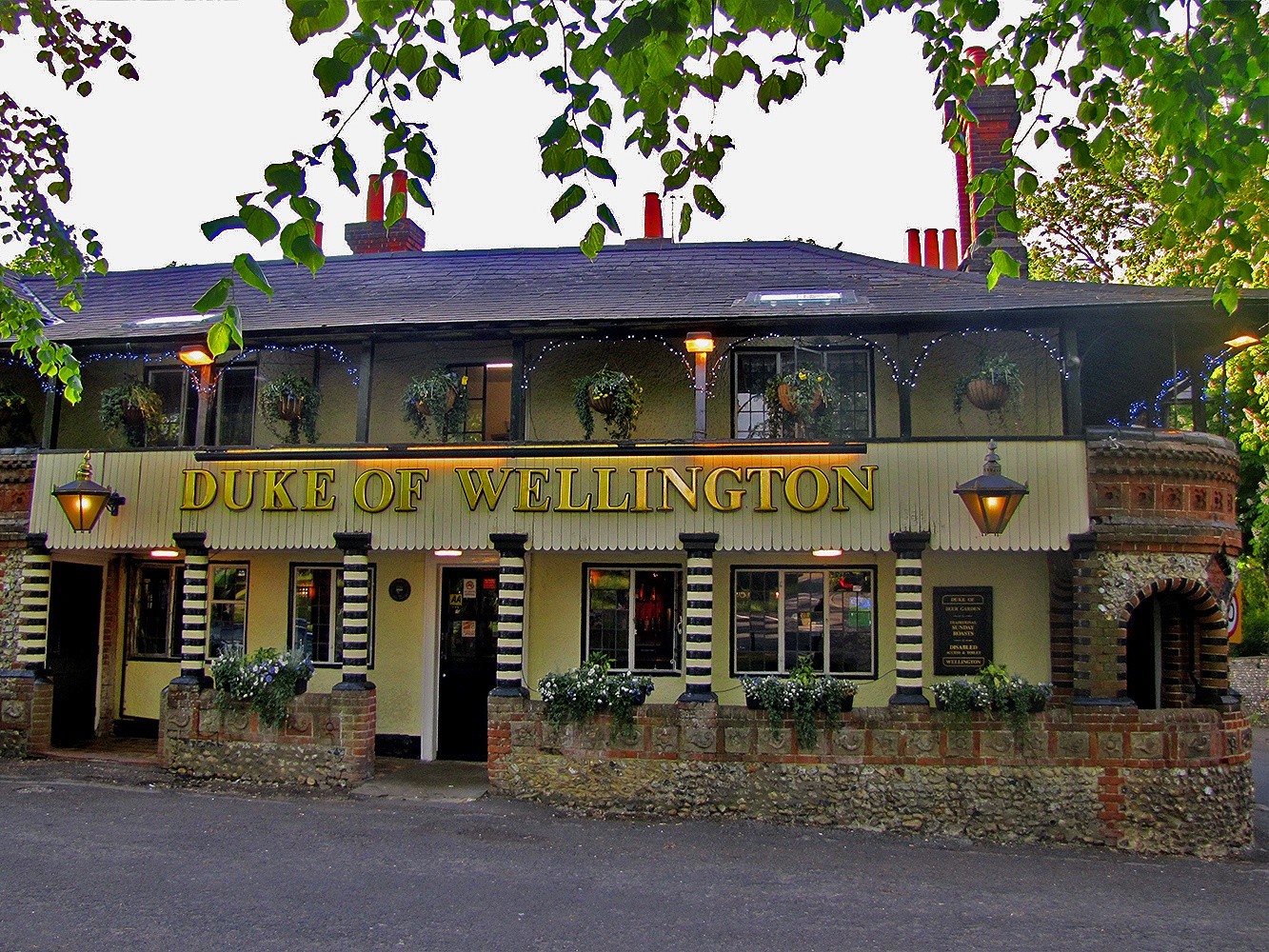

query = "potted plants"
98;373;163;442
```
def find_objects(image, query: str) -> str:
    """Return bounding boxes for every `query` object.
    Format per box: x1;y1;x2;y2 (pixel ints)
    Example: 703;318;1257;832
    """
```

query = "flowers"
764;363;843;444
537;651;1055;752
257;372;323;444
208;641;314;728
952;350;1025;438
0;385;27;439
399;368;469;443
572;369;645;440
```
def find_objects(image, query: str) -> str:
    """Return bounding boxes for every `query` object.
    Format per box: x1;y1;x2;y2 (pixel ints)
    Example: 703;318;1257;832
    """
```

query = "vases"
777;384;823;414
275;396;304;421
934;692;1047;713
746;695;854;712
416;387;456;415
215;676;307;693
588;386;616;413
632;686;647;706
966;378;1009;410
0;409;10;425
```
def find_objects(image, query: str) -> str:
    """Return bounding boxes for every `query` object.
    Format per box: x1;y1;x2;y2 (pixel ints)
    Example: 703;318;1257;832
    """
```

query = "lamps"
684;331;715;353
51;450;125;533
953;439;1029;536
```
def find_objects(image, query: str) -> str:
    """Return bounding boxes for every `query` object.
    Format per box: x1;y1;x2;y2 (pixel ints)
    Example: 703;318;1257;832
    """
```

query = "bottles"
645;616;650;631
639;617;643;630
651;617;655;630
